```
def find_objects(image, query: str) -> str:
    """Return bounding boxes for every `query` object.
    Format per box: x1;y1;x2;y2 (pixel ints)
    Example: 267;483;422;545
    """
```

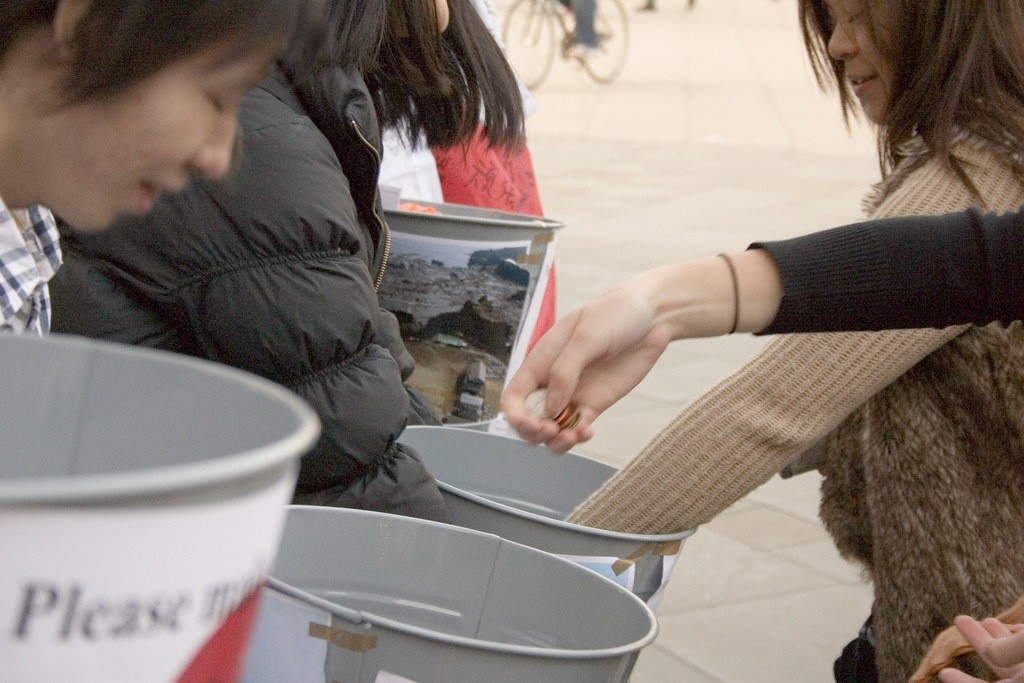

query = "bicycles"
501;0;631;92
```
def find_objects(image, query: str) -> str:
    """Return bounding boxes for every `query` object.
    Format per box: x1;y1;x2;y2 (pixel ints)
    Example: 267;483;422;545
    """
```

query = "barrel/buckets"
396;426;700;605
383;198;564;433
245;505;660;683
0;328;321;683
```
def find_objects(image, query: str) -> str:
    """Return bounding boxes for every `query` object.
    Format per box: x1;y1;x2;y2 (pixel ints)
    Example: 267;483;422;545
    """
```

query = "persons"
0;0;325;336
563;0;1024;683
48;0;524;523
939;614;1024;683
500;206;1024;455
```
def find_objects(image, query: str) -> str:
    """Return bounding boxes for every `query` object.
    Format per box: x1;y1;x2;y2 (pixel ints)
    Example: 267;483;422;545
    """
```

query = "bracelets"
718;252;739;335
